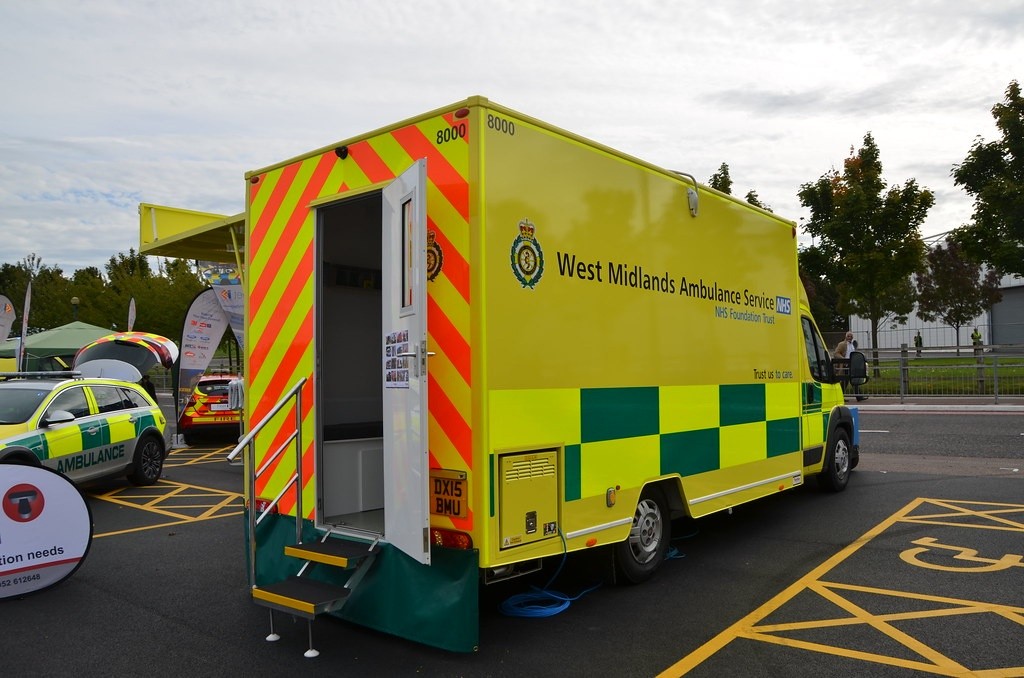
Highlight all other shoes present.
[857,397,868,401]
[844,399,849,402]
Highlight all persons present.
[914,332,923,357]
[970,328,983,358]
[142,374,158,405]
[834,331,868,403]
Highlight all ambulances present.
[181,375,243,443]
[0,371,174,486]
[137,95,870,660]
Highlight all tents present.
[0,320,124,379]
